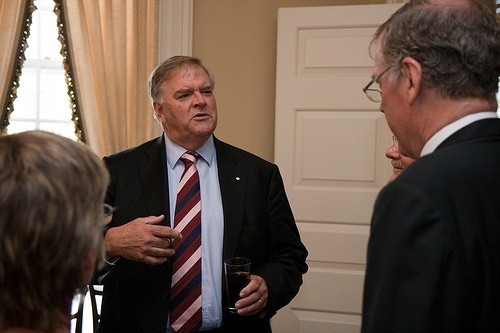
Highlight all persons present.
[385,133,417,176]
[96,56,308,333]
[0,129,114,333]
[361,0,500,333]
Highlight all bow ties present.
[165,152,203,333]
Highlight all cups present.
[224,257,251,314]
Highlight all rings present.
[166,237,173,248]
[258,299,263,305]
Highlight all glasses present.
[103,203,117,226]
[363,62,396,102]
